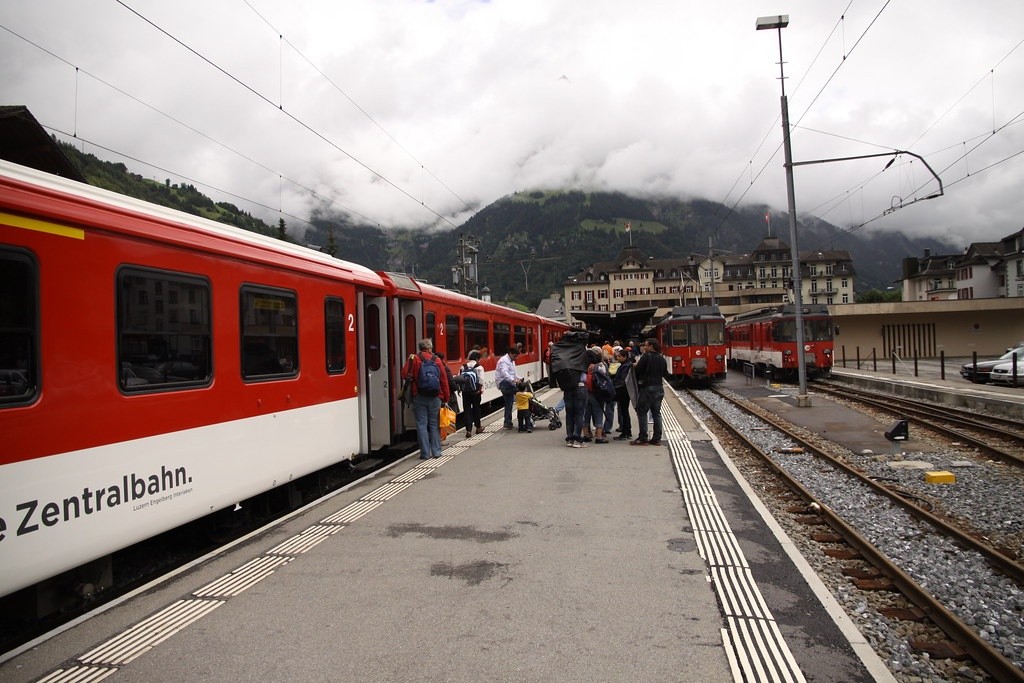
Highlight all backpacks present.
[460,363,483,397]
[417,354,441,398]
[592,364,616,403]
[543,347,551,363]
[554,365,581,391]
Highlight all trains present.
[0,158,579,618]
[642,303,728,384]
[724,302,841,382]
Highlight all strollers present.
[513,378,562,431]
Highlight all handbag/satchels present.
[398,354,414,405]
[439,402,457,433]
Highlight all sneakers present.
[567,440,573,446]
[573,440,591,448]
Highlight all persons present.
[515,382,533,433]
[458,351,485,438]
[551,327,593,449]
[400,338,450,460]
[467,340,531,359]
[613,349,635,441]
[590,339,641,365]
[635,342,657,425]
[494,347,522,430]
[434,351,456,448]
[580,346,611,444]
[544,341,554,384]
[629,339,668,447]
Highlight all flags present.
[765,212,769,222]
[625,223,631,232]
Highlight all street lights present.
[753,15,813,409]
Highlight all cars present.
[989,355,1024,385]
[959,343,1024,384]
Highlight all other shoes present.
[503,426,515,430]
[595,438,609,443]
[518,427,527,433]
[604,431,611,434]
[526,428,532,433]
[440,441,449,446]
[566,432,593,442]
[595,431,607,437]
[630,438,649,447]
[645,437,661,446]
[476,427,485,434]
[616,427,623,432]
[466,430,472,438]
[613,434,632,440]
